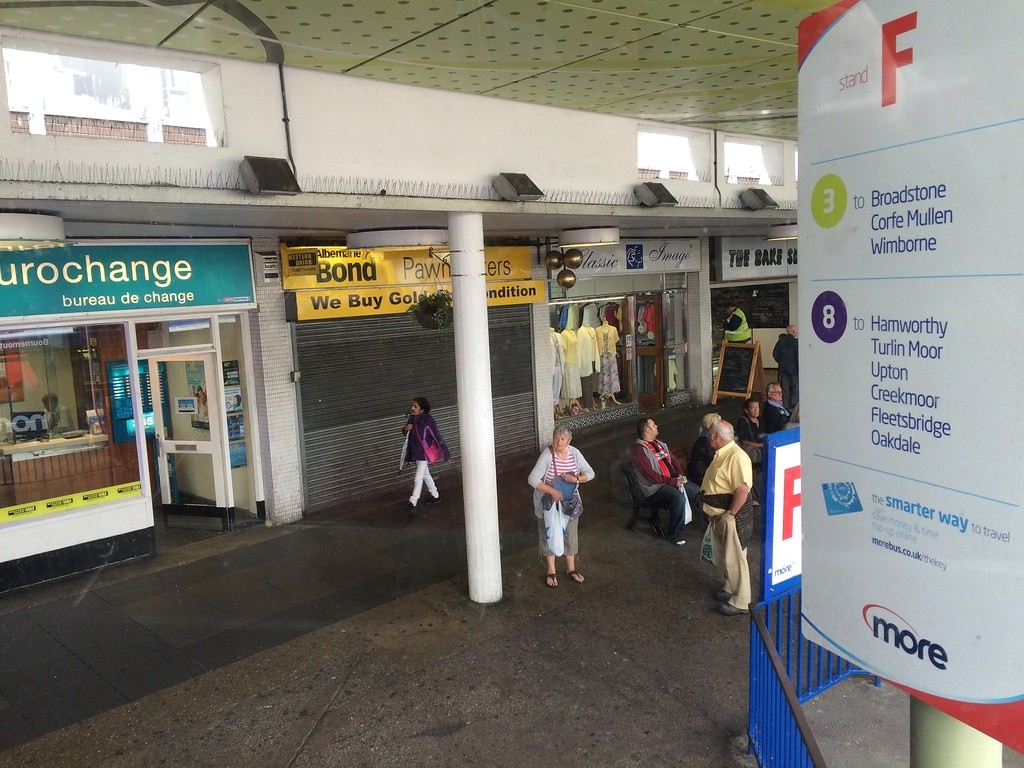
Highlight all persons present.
[551,320,622,418]
[632,417,703,546]
[190,385,209,423]
[691,383,799,506]
[718,301,753,345]
[528,424,595,588]
[695,419,756,615]
[772,325,798,412]
[401,398,452,512]
[227,395,241,410]
[41,393,71,434]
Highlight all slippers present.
[546,573,559,588]
[564,570,585,584]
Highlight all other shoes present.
[406,501,419,515]
[423,496,442,506]
[669,533,687,547]
[713,590,732,601]
[718,603,750,615]
[692,519,709,530]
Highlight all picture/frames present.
[176,398,198,414]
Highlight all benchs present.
[623,440,762,539]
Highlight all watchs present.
[576,477,580,484]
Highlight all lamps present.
[491,171,547,201]
[240,154,302,199]
[767,223,798,242]
[739,188,781,211]
[634,182,680,209]
[557,227,622,248]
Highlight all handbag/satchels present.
[679,485,692,525]
[542,501,570,557]
[699,522,715,566]
[541,471,584,521]
[424,441,442,465]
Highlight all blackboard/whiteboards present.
[713,340,765,397]
[710,282,790,329]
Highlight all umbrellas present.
[398,413,414,475]
